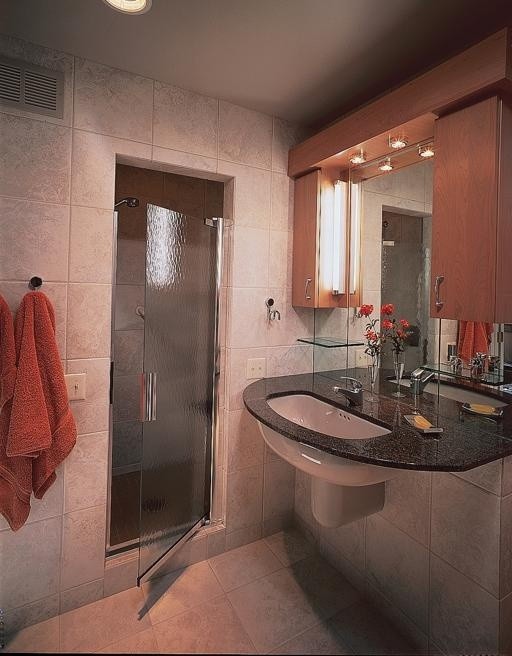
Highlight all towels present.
[0,293,78,532]
[454,320,494,364]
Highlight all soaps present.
[470,403,492,414]
[414,415,431,428]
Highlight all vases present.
[391,351,408,399]
[365,350,382,398]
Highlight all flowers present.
[356,305,393,389]
[381,305,410,390]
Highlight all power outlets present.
[248,358,266,379]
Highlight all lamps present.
[348,152,366,166]
[419,145,435,159]
[377,161,394,173]
[333,178,343,295]
[387,136,408,150]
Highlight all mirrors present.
[314,135,512,401]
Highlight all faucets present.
[333,376,364,406]
[409,361,435,395]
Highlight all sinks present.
[385,366,512,410]
[265,389,394,441]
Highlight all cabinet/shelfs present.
[293,170,348,307]
[427,95,512,323]
